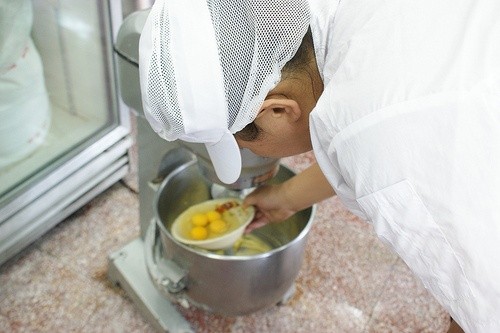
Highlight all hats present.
[138,0,311,185]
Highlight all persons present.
[137,0,496,332]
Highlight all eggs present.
[177,205,241,242]
